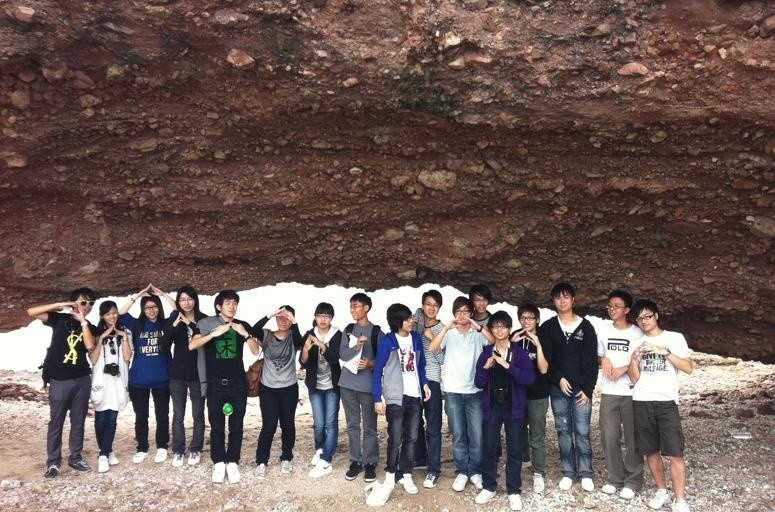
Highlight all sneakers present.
[522,461,532,468]
[559,477,573,490]
[132,451,149,464]
[211,462,226,483]
[647,489,671,509]
[452,473,468,491]
[280,460,293,477]
[671,498,690,512]
[43,464,59,480]
[413,460,427,470]
[619,486,636,499]
[311,448,323,465]
[386,471,396,489]
[226,462,241,484]
[254,463,266,481]
[109,452,120,466]
[154,448,168,463]
[345,463,362,481]
[309,460,332,478]
[364,463,377,482]
[423,473,440,488]
[98,456,109,473]
[507,494,523,510]
[398,473,419,495]
[172,452,184,468]
[187,451,201,466]
[68,459,91,473]
[601,484,617,494]
[470,474,483,489]
[532,472,545,494]
[581,478,594,492]
[475,489,497,504]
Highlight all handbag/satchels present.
[244,358,264,397]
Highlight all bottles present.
[223,403,233,416]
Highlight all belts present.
[211,378,244,385]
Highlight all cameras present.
[104,363,120,375]
[492,385,509,403]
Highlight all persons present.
[300,282,692,512]
[27,285,259,484]
[252,305,304,477]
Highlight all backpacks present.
[42,347,50,385]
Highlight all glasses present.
[349,305,364,310]
[74,300,95,306]
[520,317,536,321]
[455,310,470,314]
[178,298,194,303]
[405,315,412,322]
[143,305,159,311]
[489,325,506,329]
[635,313,654,322]
[424,303,439,308]
[606,304,627,310]
[109,341,116,355]
[315,315,330,318]
